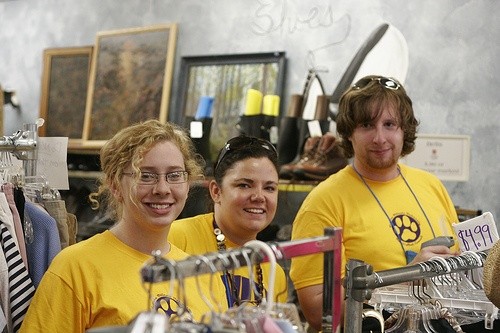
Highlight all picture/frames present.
[38,24,177,148]
[176,51,287,171]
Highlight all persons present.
[168,135,289,325]
[15,120,229,333]
[289,75,500,333]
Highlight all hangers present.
[0,135,62,201]
[353,248,499,333]
[88,240,295,333]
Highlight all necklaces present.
[213,221,263,306]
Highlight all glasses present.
[122,170,189,185]
[356,76,400,91]
[214,138,278,170]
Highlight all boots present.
[287,133,350,180]
[277,136,322,180]
